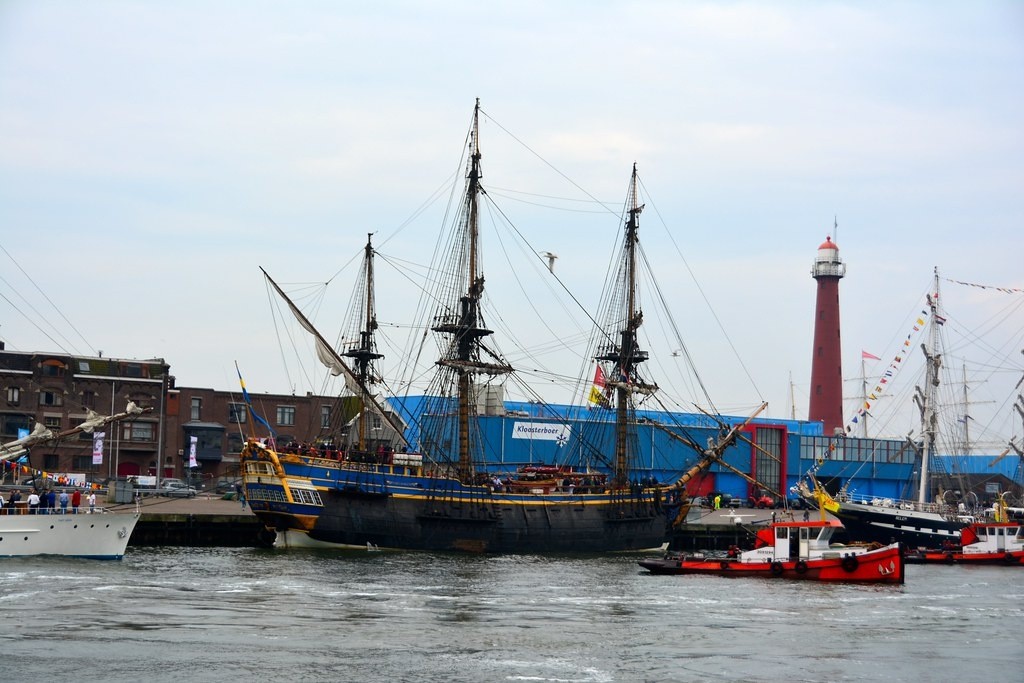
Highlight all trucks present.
[36,472,87,486]
[128,473,163,489]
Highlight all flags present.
[594,364,614,398]
[590,387,611,410]
[0,457,106,489]
[790,278,1023,492]
[620,363,631,384]
[92,431,105,464]
[189,436,198,468]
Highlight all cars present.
[23,475,42,485]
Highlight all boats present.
[636,521,907,585]
[852,521,1024,568]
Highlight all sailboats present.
[235,98,770,552]
[0,363,155,562]
[789,264,1024,547]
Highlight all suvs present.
[160,477,197,498]
[216,480,232,494]
[101,476,127,488]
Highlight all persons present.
[267,435,669,494]
[0,486,97,514]
[803,508,810,522]
[729,507,736,522]
[714,494,720,510]
[241,491,248,511]
[726,544,740,559]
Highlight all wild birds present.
[538,250,557,274]
[669,349,682,357]
